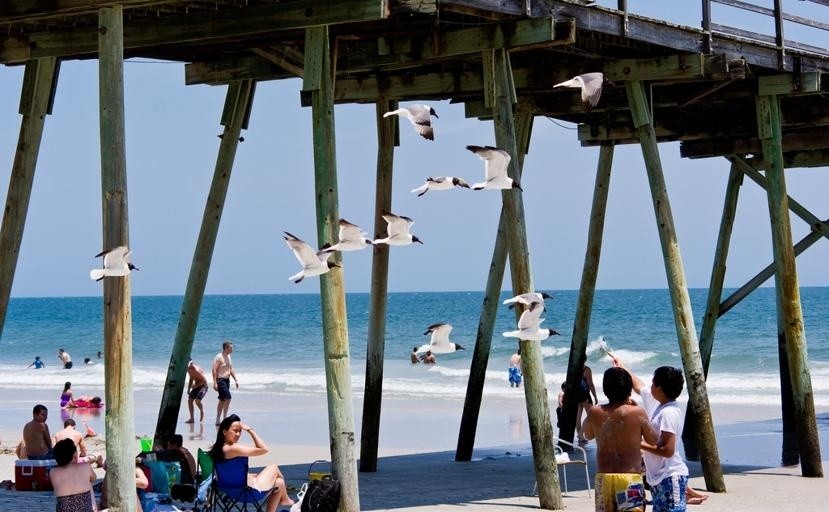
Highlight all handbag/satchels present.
[301,475,340,512]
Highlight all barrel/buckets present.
[141,439,151,452]
[308,460,332,481]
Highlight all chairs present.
[210,458,280,512]
[532,435,593,501]
[195,448,241,511]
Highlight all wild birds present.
[553,71,616,107]
[502,306,561,342]
[413,324,466,361]
[322,218,377,252]
[89,245,140,282]
[465,145,523,192]
[373,209,423,246]
[503,292,553,313]
[280,230,342,286]
[410,176,470,197]
[383,104,439,141]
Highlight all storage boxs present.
[14,460,56,492]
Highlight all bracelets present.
[246,427,252,434]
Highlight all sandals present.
[156,497,171,504]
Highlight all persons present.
[507,349,523,387]
[557,348,690,511]
[211,340,239,426]
[22,348,196,511]
[186,359,208,424]
[413,348,435,364]
[206,414,295,512]
